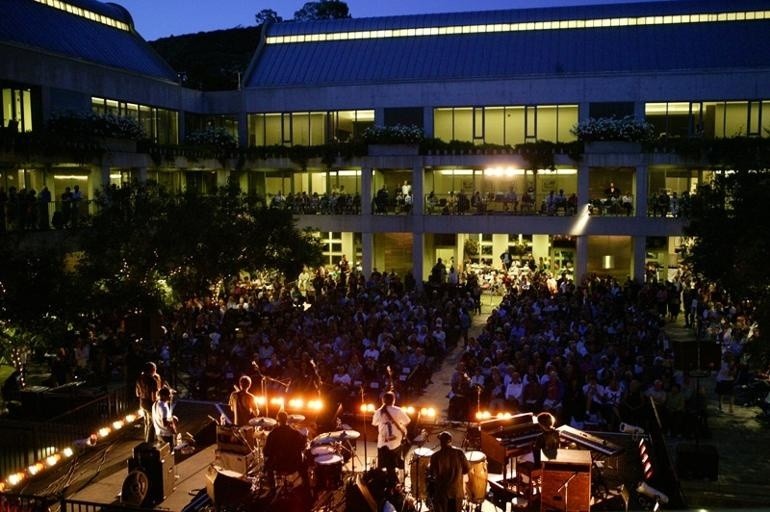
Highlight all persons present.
[537,413,561,460]
[371,392,411,470]
[52,255,770,431]
[61,185,82,210]
[136,362,161,443]
[275,184,361,216]
[7,186,51,229]
[590,182,634,217]
[263,413,309,495]
[376,181,412,215]
[648,189,690,218]
[150,386,177,453]
[428,185,578,216]
[427,432,469,512]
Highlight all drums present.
[410,448,433,500]
[465,451,487,504]
[315,455,342,489]
[309,445,333,486]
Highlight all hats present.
[708,301,723,307]
[437,431,453,443]
[284,283,471,331]
[482,334,663,371]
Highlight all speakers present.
[127,452,175,502]
[672,339,722,371]
[540,449,592,512]
[205,464,252,503]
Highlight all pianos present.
[479,412,546,482]
[556,426,623,456]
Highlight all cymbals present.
[288,414,305,423]
[249,417,277,426]
[338,430,359,438]
[316,433,338,443]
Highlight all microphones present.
[387,365,392,375]
[252,360,259,368]
[310,359,316,367]
[463,373,472,383]
